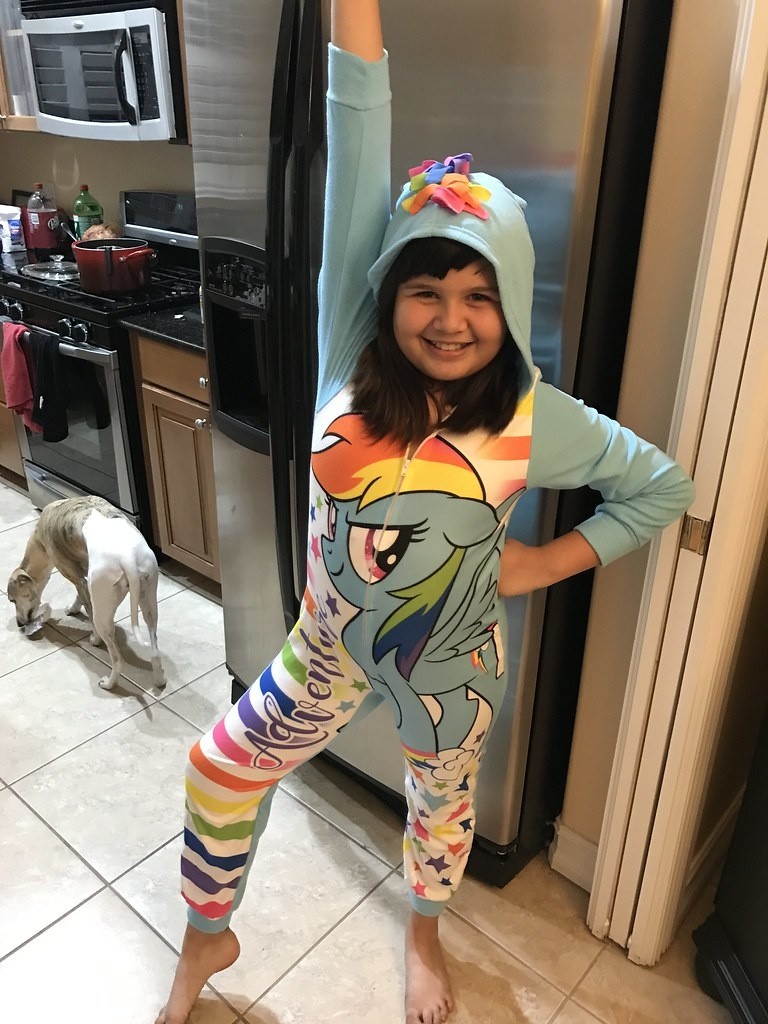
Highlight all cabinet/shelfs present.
[1,325,24,479]
[138,333,222,586]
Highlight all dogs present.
[7,495,168,690]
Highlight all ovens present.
[1,295,154,555]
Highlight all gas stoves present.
[0,253,200,327]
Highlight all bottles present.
[26,183,64,264]
[73,184,103,242]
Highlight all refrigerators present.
[181,0,672,894]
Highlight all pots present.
[71,236,155,293]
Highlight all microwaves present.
[19,6,186,141]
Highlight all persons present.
[155,0,698,1024]
